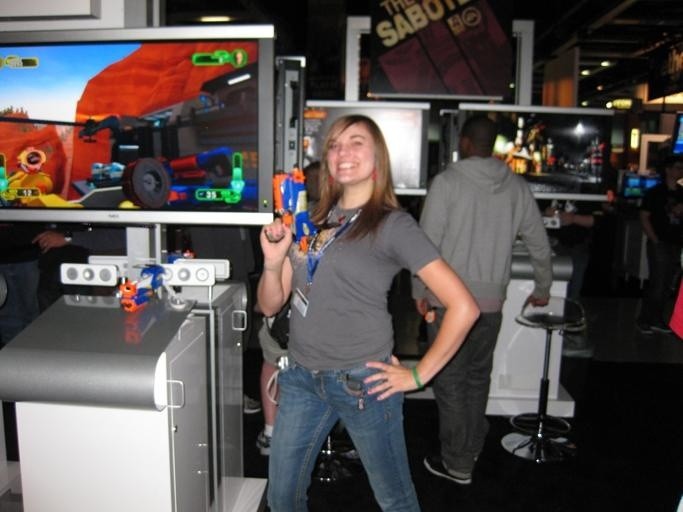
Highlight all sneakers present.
[256,432,272,456]
[636,319,651,334]
[652,320,672,333]
[424,455,472,484]
[243,394,261,413]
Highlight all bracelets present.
[411,364,423,391]
[63,231,72,245]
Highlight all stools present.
[499,293,589,465]
[267,293,362,484]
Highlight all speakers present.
[173,258,230,281]
[540,216,560,227]
[87,255,129,277]
[159,265,215,287]
[59,263,119,287]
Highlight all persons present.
[636,153,683,335]
[0,222,41,330]
[257,161,320,456]
[190,226,260,415]
[31,223,126,256]
[411,112,552,481]
[544,201,609,295]
[258,114,481,512]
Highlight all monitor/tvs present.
[671,112,683,158]
[458,103,615,202]
[0,25,275,224]
[301,100,432,195]
[273,57,306,214]
